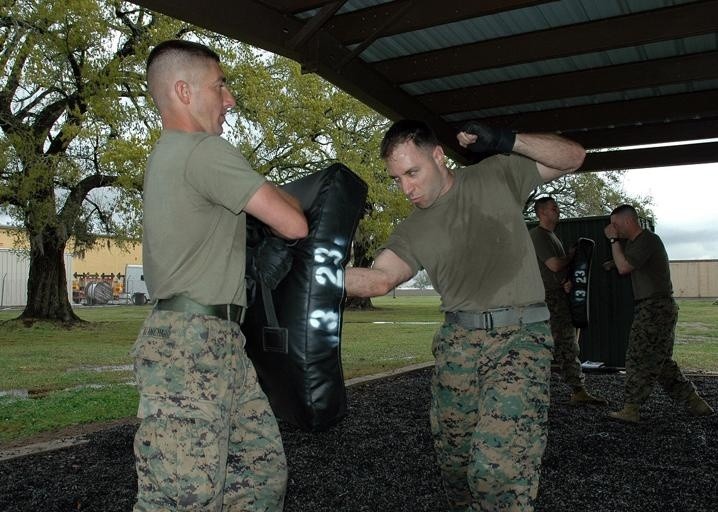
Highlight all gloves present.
[252,237,294,291]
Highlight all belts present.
[157,297,243,324]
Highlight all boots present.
[686,390,714,416]
[609,404,642,422]
[571,384,608,405]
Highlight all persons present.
[131,40,309,512]
[343,120,585,512]
[527,197,610,408]
[604,205,713,425]
